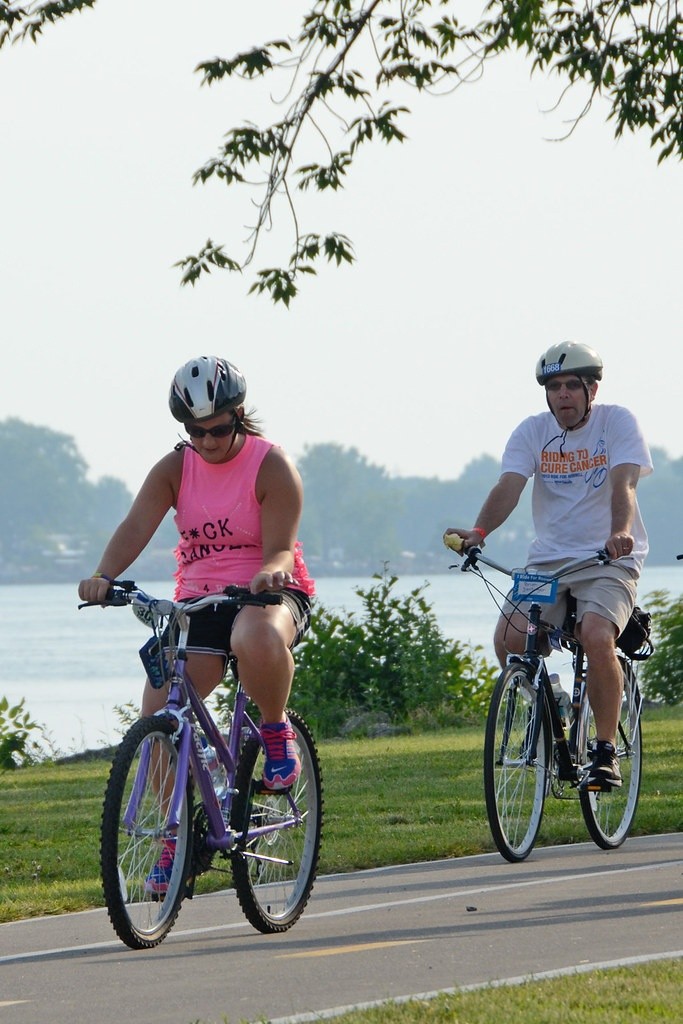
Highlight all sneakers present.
[143,832,178,895]
[518,705,537,765]
[587,737,622,787]
[259,710,302,790]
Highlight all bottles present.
[548,673,575,730]
[200,737,228,797]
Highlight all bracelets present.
[91,573,113,582]
[471,528,486,541]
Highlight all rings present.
[623,547,629,550]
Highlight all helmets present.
[169,356,247,424]
[536,345,603,386]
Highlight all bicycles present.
[77,580,326,951]
[447,538,647,864]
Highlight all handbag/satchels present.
[616,605,655,661]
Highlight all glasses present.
[184,413,236,439]
[545,379,590,392]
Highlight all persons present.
[79,357,318,895]
[442,340,654,789]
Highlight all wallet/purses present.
[139,636,172,690]
